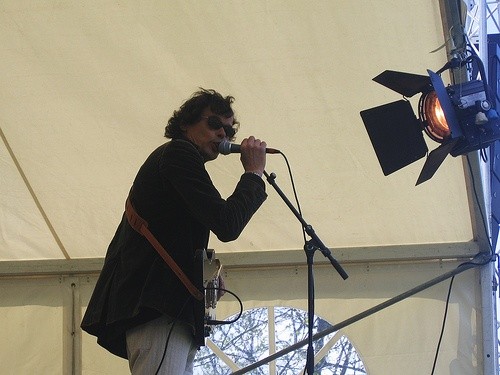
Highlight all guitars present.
[192,247,224,346]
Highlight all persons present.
[79,87,268,375]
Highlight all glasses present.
[202,115,235,138]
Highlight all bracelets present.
[244,170,260,178]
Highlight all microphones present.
[218,141,280,155]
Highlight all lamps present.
[359,55,500,186]
